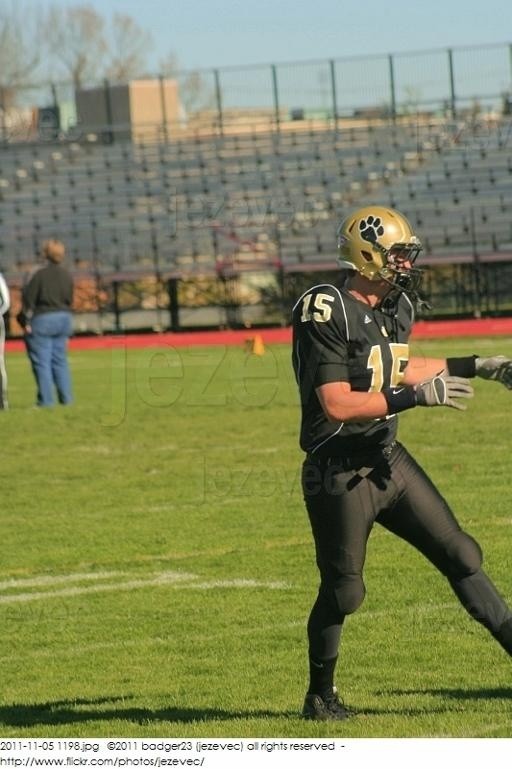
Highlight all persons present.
[0,272,12,409]
[292,205,511,723]
[13,239,76,407]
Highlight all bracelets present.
[379,384,417,415]
[446,354,480,379]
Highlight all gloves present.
[414,367,474,411]
[474,355,512,389]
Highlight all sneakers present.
[301,687,357,721]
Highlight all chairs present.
[0,115,510,274]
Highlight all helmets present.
[336,206,425,293]
